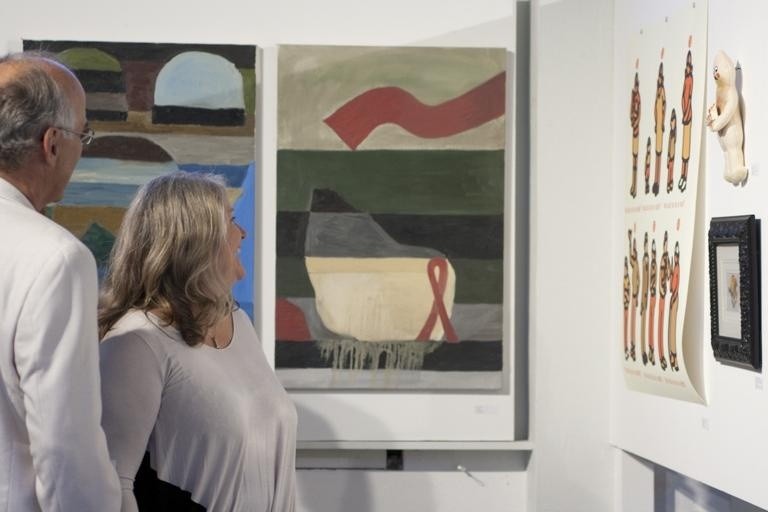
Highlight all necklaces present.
[204,325,220,350]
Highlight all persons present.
[97,174,300,512]
[1,49,122,512]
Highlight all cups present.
[54,126,95,147]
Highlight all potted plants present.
[708,213,762,371]
